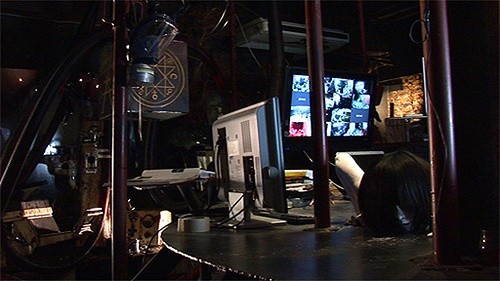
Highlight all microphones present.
[424,7,430,33]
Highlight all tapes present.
[177,216,210,232]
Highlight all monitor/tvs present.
[212,97,288,215]
[281,64,379,150]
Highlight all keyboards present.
[267,211,315,225]
